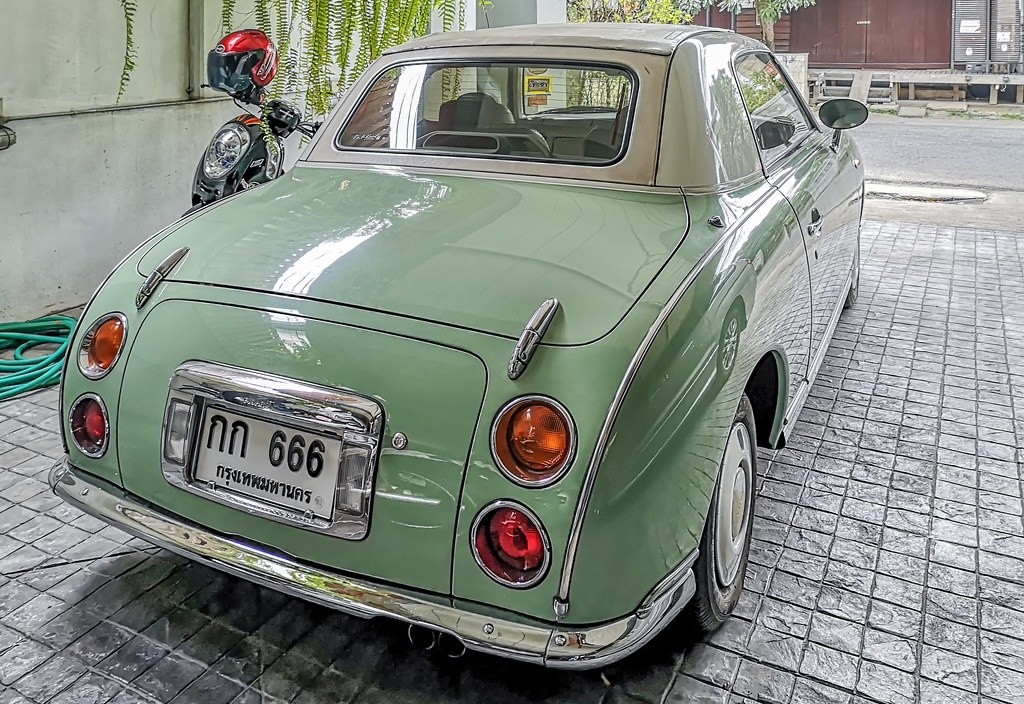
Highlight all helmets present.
[215,29,279,87]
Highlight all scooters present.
[183,75,329,218]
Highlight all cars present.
[45,22,870,672]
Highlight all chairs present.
[585,106,629,159]
[440,92,527,151]
